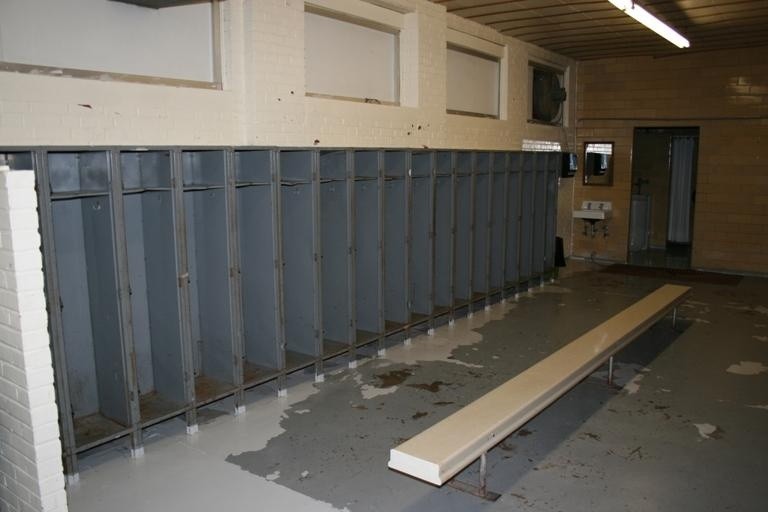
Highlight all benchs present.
[388,283,692,502]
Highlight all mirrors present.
[583,141,614,186]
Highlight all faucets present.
[586,203,592,209]
[598,203,604,209]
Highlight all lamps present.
[610,0,691,49]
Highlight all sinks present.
[572,208,612,219]
